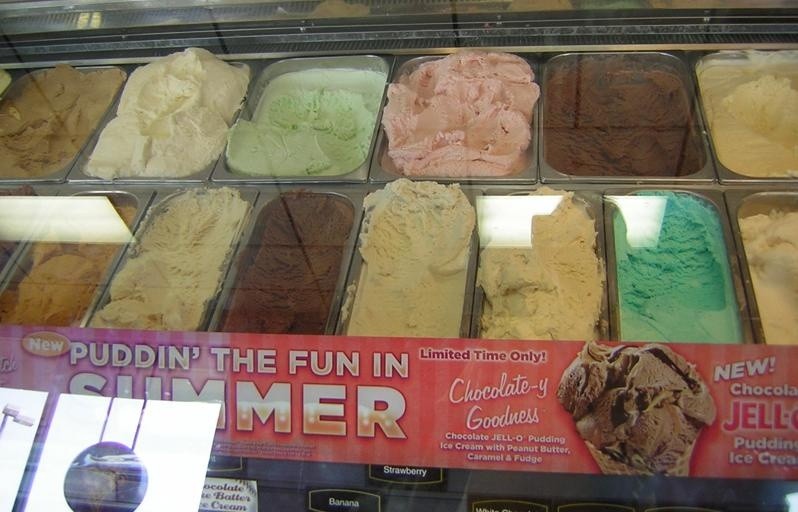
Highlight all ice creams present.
[555,339,716,477]
